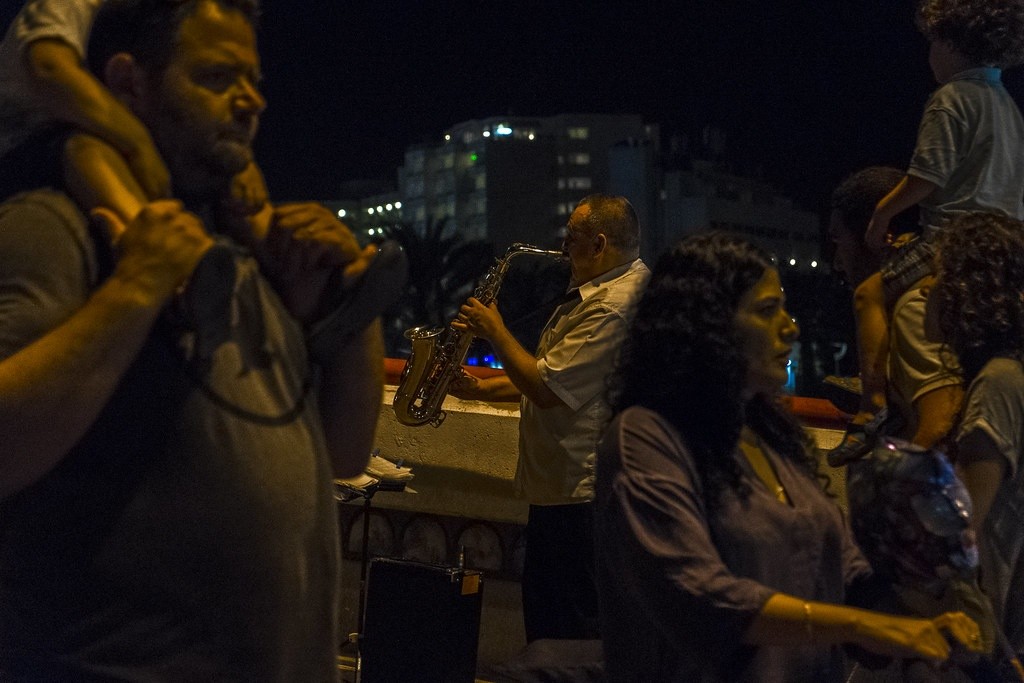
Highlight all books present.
[330,453,418,498]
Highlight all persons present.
[446,0,1024,683]
[0,0,382,683]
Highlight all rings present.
[968,634,979,644]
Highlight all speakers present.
[357,557,484,683]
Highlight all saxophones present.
[390,242,572,428]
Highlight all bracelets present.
[804,599,814,648]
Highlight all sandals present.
[821,375,869,415]
[827,407,890,468]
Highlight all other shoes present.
[301,241,407,357]
[166,244,238,371]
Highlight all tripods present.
[331,481,407,683]
